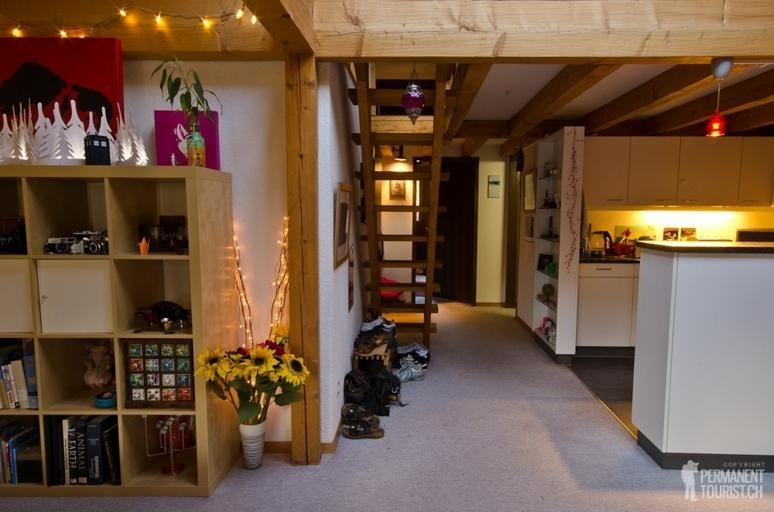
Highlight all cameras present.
[72,231,102,252]
[47,238,76,254]
[84,239,108,255]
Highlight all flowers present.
[194,323,310,424]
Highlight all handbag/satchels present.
[344,367,400,409]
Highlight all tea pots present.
[588,230,613,257]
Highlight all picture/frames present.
[390,180,405,200]
[333,182,354,271]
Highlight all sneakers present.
[392,342,430,383]
[354,316,397,354]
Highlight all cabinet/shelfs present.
[577,264,639,347]
[584,137,774,206]
[0,165,241,496]
[535,171,560,314]
[516,240,535,330]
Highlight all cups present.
[164,321,175,333]
[176,316,191,330]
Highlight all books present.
[0,338,39,410]
[0,415,121,488]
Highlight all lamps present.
[12,3,257,37]
[706,56,735,137]
[392,145,407,161]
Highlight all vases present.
[238,419,272,468]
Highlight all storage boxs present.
[0,37,125,141]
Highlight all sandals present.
[340,403,384,439]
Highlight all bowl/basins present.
[93,393,116,408]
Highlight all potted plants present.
[151,55,223,167]
